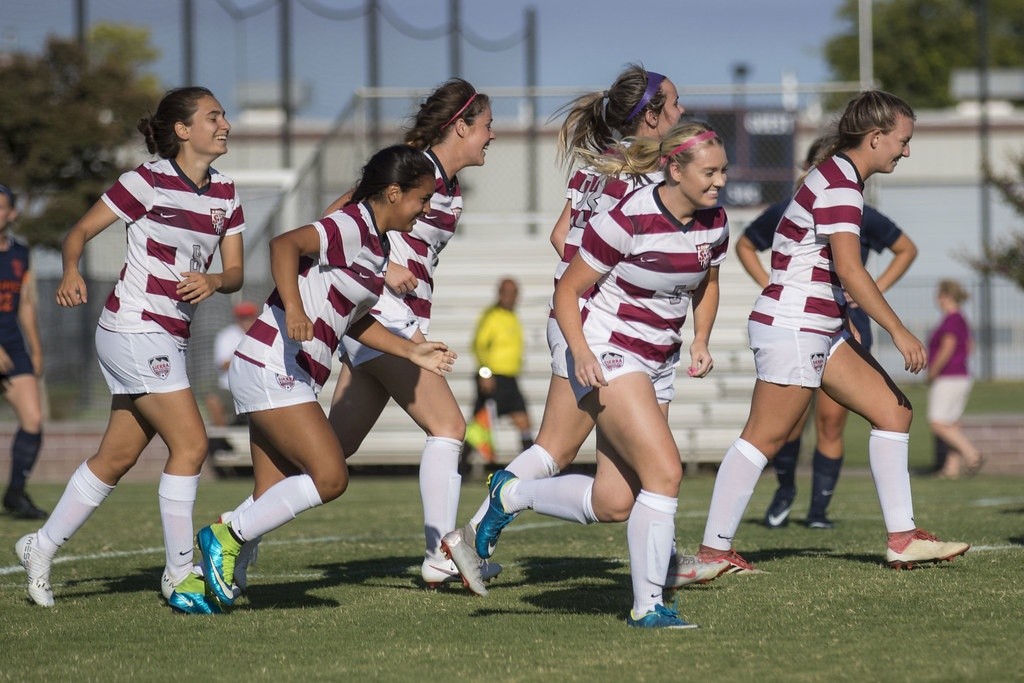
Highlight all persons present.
[477,118,729,633]
[698,90,969,570]
[738,137,916,529]
[923,281,983,480]
[197,145,457,609]
[439,65,731,596]
[205,303,261,426]
[323,77,501,589]
[15,86,246,609]
[0,184,48,519]
[455,278,535,473]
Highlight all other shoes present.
[764,485,796,529]
[13,533,55,609]
[3,491,47,519]
[160,565,206,600]
[806,508,834,531]
[927,469,960,483]
[965,453,985,478]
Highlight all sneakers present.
[169,573,253,614]
[662,539,731,591]
[422,556,502,589]
[699,549,772,576]
[887,528,971,569]
[473,469,518,559]
[626,595,698,630]
[439,526,489,597]
[195,523,242,606]
[218,511,259,592]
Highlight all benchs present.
[198,207,771,467]
